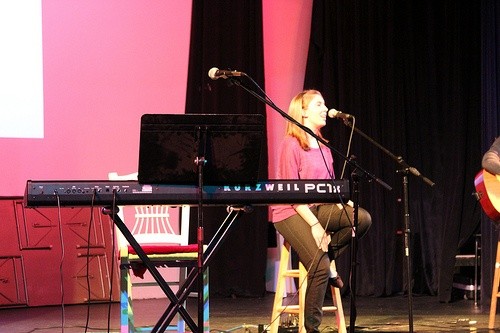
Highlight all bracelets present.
[310,221,320,227]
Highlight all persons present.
[269,90,372,333]
[481,136,500,175]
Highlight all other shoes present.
[329,274,343,287]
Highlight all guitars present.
[474,168,500,220]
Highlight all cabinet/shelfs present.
[453,240,481,310]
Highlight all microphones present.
[328,109,353,118]
[208,67,243,80]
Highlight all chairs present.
[109,171,210,333]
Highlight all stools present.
[270,232,347,333]
[488,241,500,329]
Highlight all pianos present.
[22,178,350,208]
